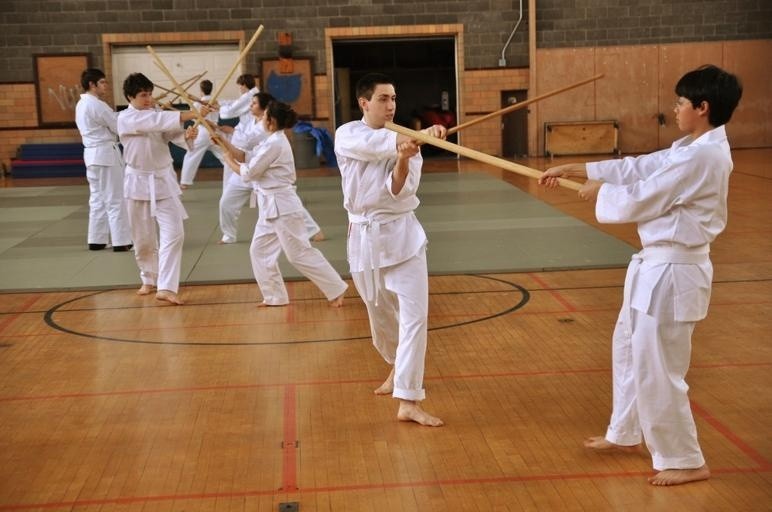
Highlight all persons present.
[209,98,350,309]
[216,73,260,194]
[218,91,327,243]
[333,67,449,428]
[537,64,745,487]
[178,79,231,189]
[74,67,134,252]
[501,97,526,160]
[115,72,200,306]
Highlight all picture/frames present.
[31,52,92,129]
[260,56,316,121]
